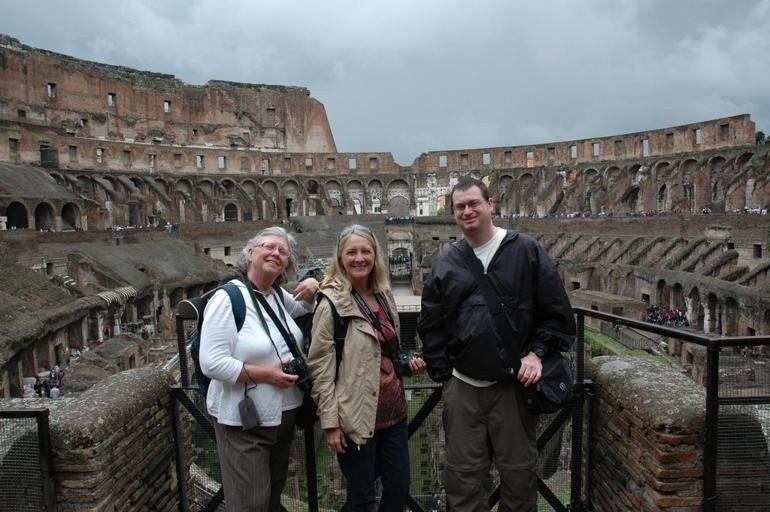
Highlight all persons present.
[643,301,690,328]
[307,223,430,512]
[33,304,165,398]
[414,177,578,510]
[197,226,321,510]
[106,220,181,241]
[491,205,770,221]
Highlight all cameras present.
[281,355,310,383]
[393,351,421,377]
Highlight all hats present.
[177,284,265,331]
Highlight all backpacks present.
[189,280,285,398]
[302,292,401,389]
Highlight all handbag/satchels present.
[515,351,572,415]
[294,395,320,430]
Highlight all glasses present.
[342,248,373,256]
[453,198,486,211]
[254,242,290,257]
